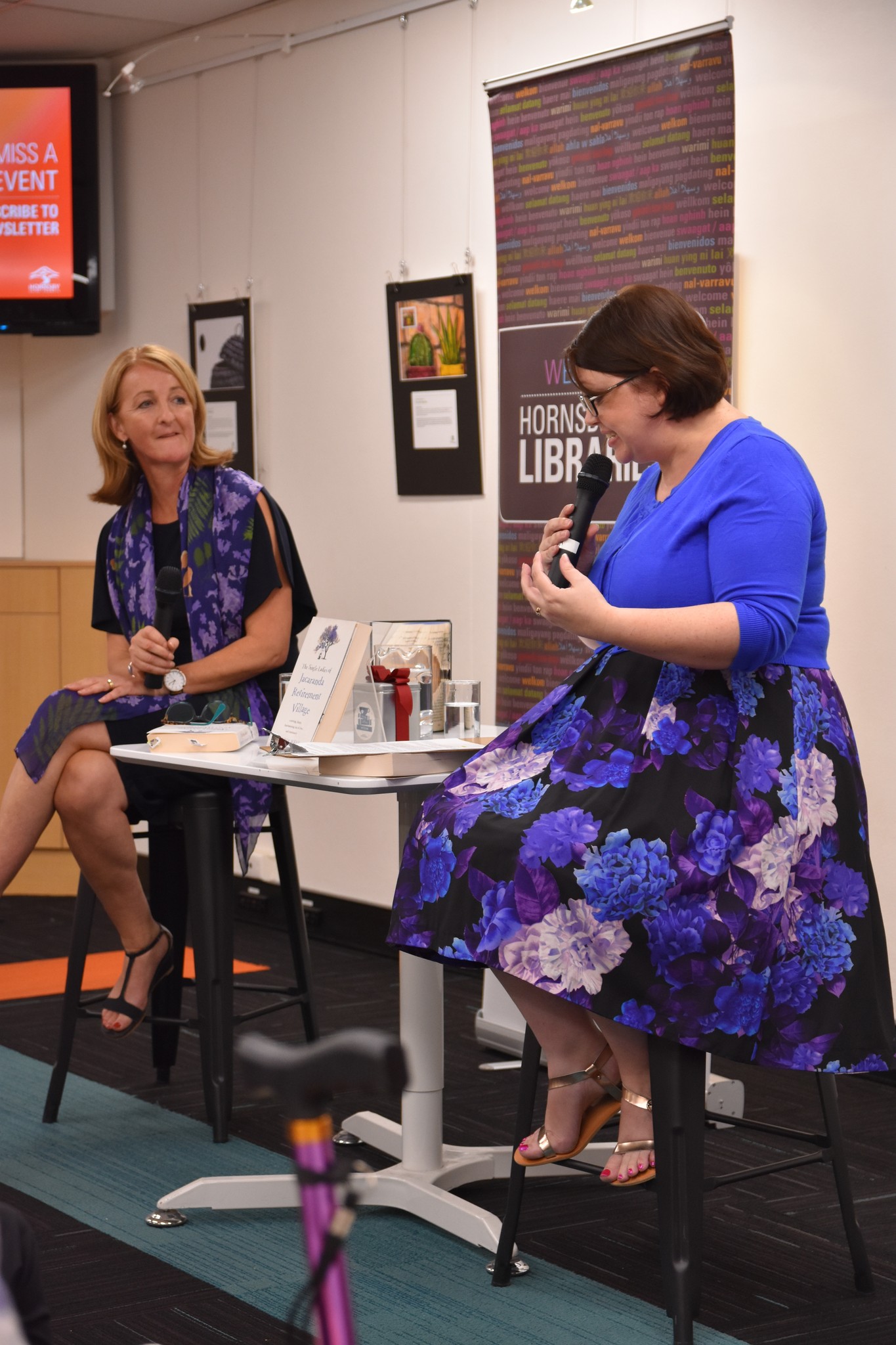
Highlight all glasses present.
[578,370,642,418]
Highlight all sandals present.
[514,1042,622,1166]
[609,1084,656,1186]
[101,921,174,1041]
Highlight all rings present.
[107,679,114,690]
[536,608,541,616]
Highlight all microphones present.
[546,453,613,589]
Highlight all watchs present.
[163,666,186,696]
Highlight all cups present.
[444,681,482,738]
[279,673,295,707]
[375,644,434,740]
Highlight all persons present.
[388,283,896,1186]
[1,345,319,1036]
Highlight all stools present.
[43,775,326,1146]
[492,1045,872,1344]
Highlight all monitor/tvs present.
[0,62,102,335]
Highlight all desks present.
[108,728,618,1277]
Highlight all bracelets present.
[127,661,135,677]
